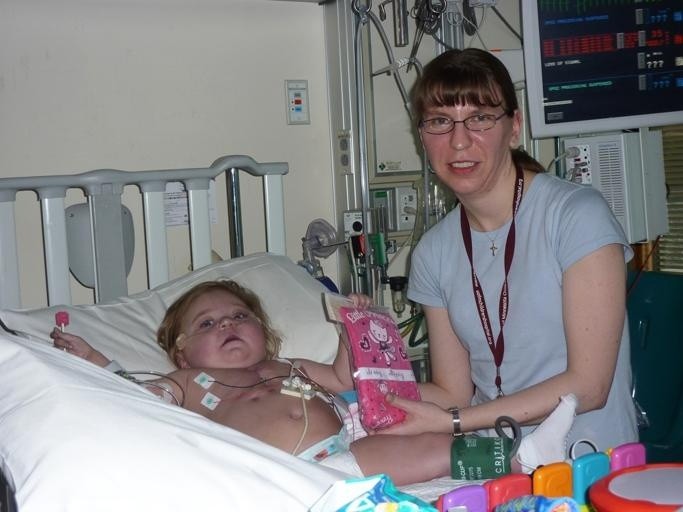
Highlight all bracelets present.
[446,405,463,439]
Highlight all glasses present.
[416,105,511,133]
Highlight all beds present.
[1,154,628,511]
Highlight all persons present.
[48,282,576,487]
[362,47,639,460]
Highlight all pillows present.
[1,254,341,382]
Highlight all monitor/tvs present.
[520,0,683,139]
